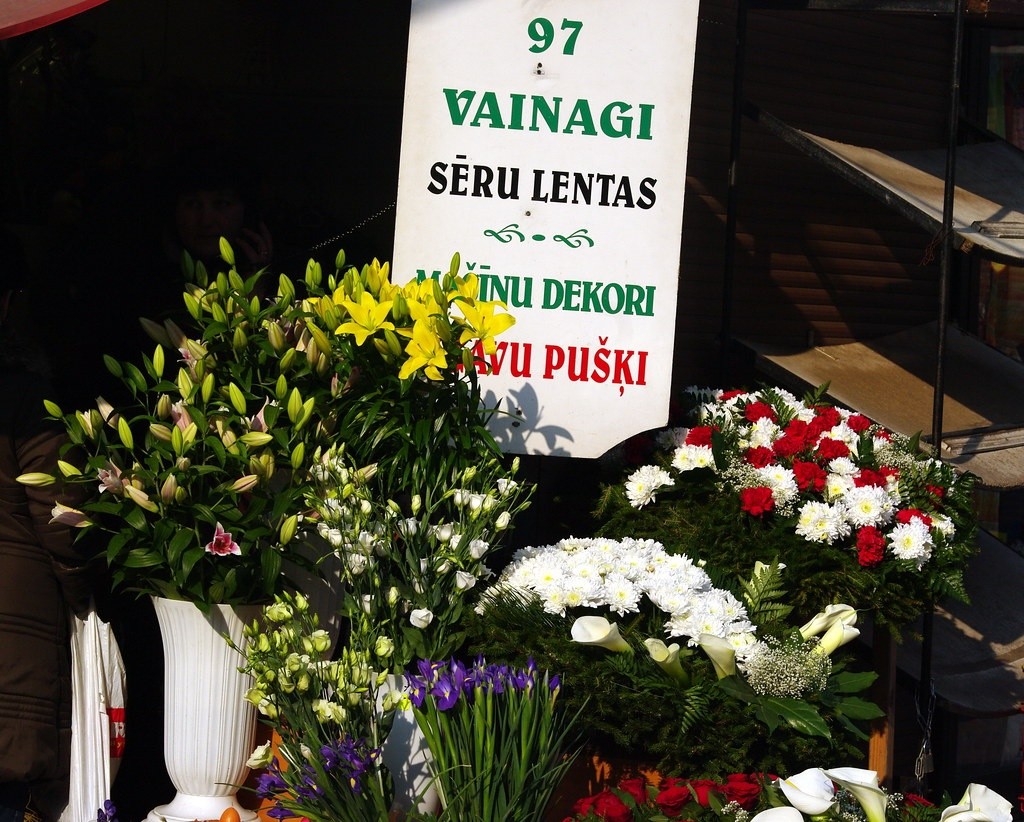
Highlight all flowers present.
[13,233,982,822]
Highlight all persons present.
[121,141,306,360]
[0,226,93,821]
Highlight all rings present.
[259,249,268,255]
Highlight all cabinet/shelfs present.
[710,0,1024,811]
